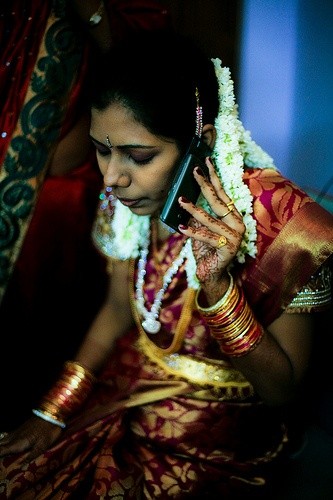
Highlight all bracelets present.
[193,272,265,358]
[32,360,100,429]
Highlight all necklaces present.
[123,240,193,358]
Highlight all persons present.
[0,23,333,500]
[75,1,110,52]
[15,108,104,314]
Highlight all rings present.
[222,199,236,218]
[216,235,227,250]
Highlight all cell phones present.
[160,135,214,233]
